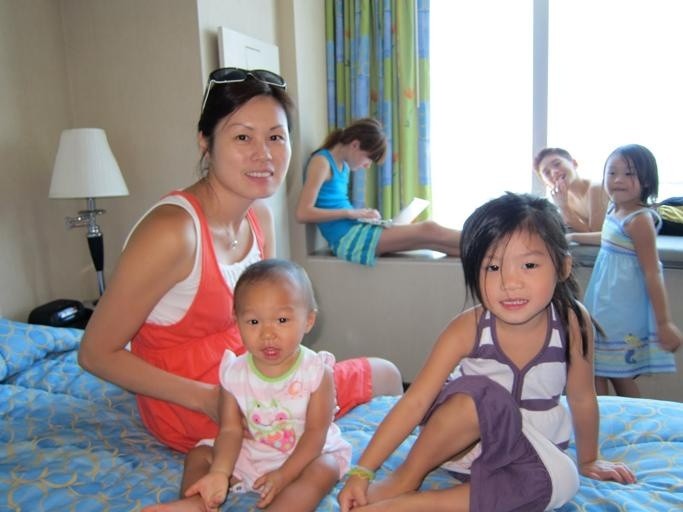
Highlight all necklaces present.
[225,220,242,251]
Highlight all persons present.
[77,67,405,453]
[337,192,636,512]
[533,147,682,236]
[140,257,352,512]
[564,143,682,396]
[295,117,462,266]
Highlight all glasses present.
[202,67,288,118]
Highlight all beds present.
[0,317,683,512]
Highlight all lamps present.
[49,127,131,296]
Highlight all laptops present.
[357,197,430,228]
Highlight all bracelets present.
[345,464,375,483]
